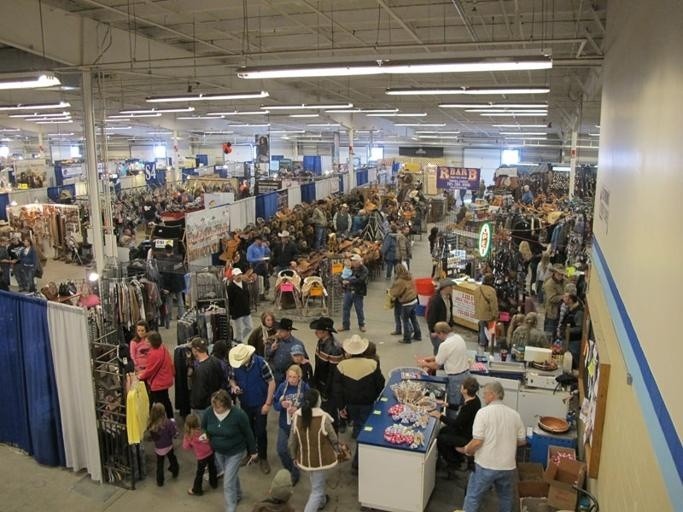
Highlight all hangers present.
[212,301,225,313]
[111,276,130,289]
[130,276,139,287]
[135,276,144,287]
[204,300,220,311]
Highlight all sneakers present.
[238,456,251,467]
[359,326,367,333]
[337,325,344,332]
[260,462,271,474]
[397,338,411,344]
[413,336,422,340]
[390,331,401,335]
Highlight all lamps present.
[324,97,401,113]
[145,78,270,104]
[438,95,549,108]
[259,78,355,110]
[385,74,550,95]
[0,93,71,110]
[0,0,61,88]
[236,0,553,80]
[119,77,194,114]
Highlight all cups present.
[500,349,508,361]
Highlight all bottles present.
[511,343,516,358]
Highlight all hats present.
[186,336,208,349]
[268,469,294,503]
[254,233,266,240]
[290,344,306,357]
[228,344,256,370]
[434,321,452,333]
[438,278,457,288]
[278,231,291,238]
[340,203,349,208]
[231,268,243,276]
[342,333,370,355]
[548,263,568,275]
[309,316,337,334]
[271,318,298,331]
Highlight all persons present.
[0,180,589,511]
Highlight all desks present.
[355,375,449,512]
[472,370,573,432]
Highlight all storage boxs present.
[520,497,556,512]
[547,445,576,465]
[516,463,548,496]
[544,458,586,511]
[526,426,578,471]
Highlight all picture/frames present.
[577,298,611,480]
